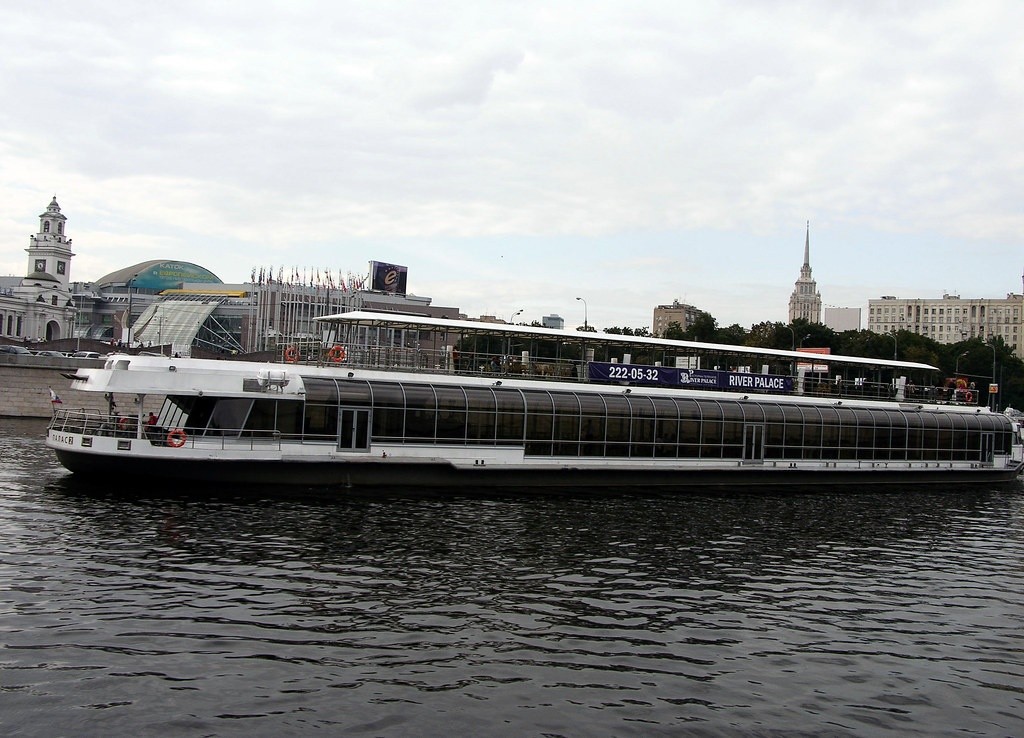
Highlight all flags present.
[49,388,61,404]
[251,264,364,294]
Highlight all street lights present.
[885,330,897,396]
[576,297,588,332]
[985,342,996,413]
[125,272,139,351]
[783,325,794,376]
[799,333,810,351]
[956,350,970,385]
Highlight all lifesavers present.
[330,346,345,362]
[167,429,187,447]
[965,392,972,402]
[285,347,299,361]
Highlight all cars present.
[0,344,167,360]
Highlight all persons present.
[146,411,157,431]
[905,379,976,403]
[22,336,48,349]
[1004,403,1014,416]
[110,338,153,347]
[452,344,578,379]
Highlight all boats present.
[39,307,1024,493]
